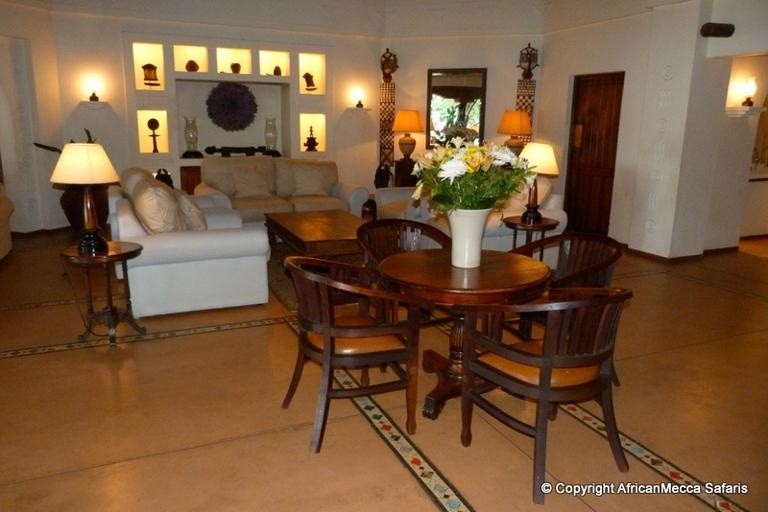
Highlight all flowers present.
[411,136,536,219]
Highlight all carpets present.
[267,243,364,312]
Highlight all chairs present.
[461,287,634,504]
[280,254,420,454]
[506,232,624,386]
[106,184,232,279]
[358,219,452,373]
[115,199,269,320]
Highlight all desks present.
[263,209,369,257]
[378,248,550,420]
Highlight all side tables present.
[61,241,146,343]
[504,216,559,262]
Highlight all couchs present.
[369,187,568,271]
[194,156,369,242]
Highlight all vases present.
[446,207,492,269]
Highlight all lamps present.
[392,110,425,161]
[379,48,399,168]
[742,76,758,106]
[496,110,533,156]
[51,143,121,254]
[515,43,539,146]
[515,142,561,222]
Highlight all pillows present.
[230,164,271,198]
[120,168,154,199]
[134,178,207,235]
[275,161,335,196]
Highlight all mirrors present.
[426,68,487,150]
